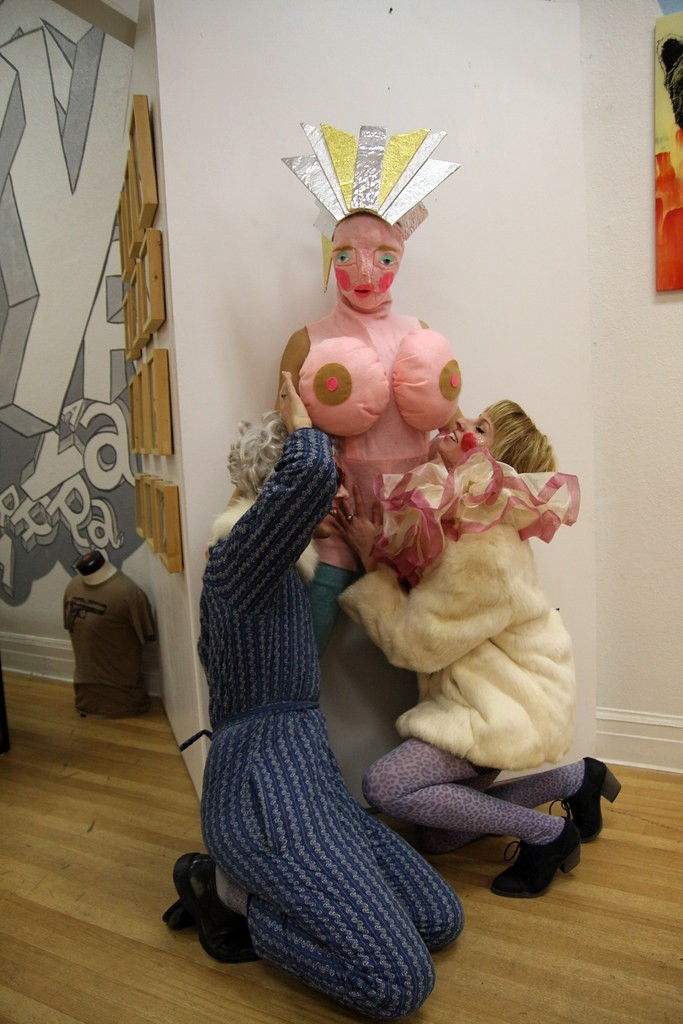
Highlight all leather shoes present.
[173,852,259,962]
[162,896,192,930]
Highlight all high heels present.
[548,757,621,842]
[491,816,581,898]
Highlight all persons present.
[57,551,158,720]
[332,398,621,896]
[162,367,465,1022]
[205,214,463,664]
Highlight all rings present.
[281,394,286,398]
[346,513,356,521]
